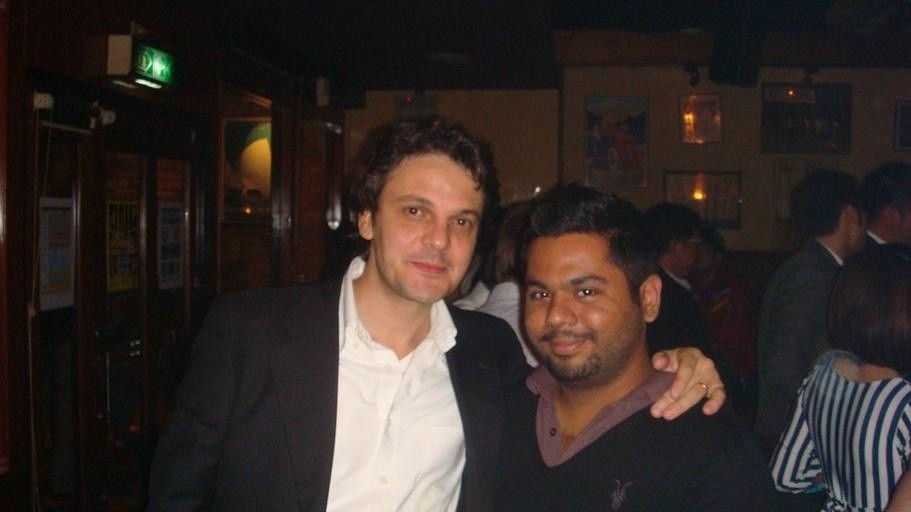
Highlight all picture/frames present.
[581,95,649,191]
[217,116,272,226]
[678,93,724,144]
[894,100,911,152]
[663,170,741,229]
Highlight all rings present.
[696,380,710,396]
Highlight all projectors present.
[391,94,439,117]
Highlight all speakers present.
[708,19,763,89]
[327,77,367,111]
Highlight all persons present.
[453,197,541,373]
[621,198,715,373]
[768,241,910,512]
[748,163,870,512]
[145,111,729,512]
[489,181,770,511]
[856,159,911,250]
[688,227,763,430]
[447,207,506,312]
